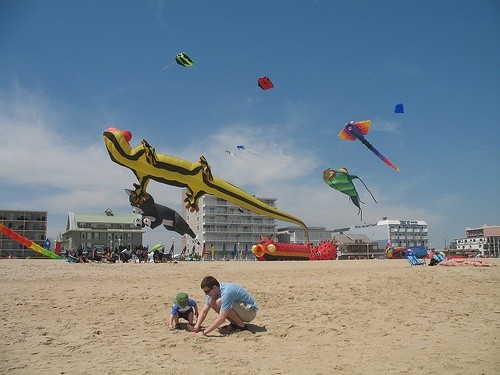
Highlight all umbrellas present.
[149,243,165,253]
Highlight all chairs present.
[407,256,426,266]
[433,254,449,265]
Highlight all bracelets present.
[202,330,206,335]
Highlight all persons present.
[169,291,198,331]
[193,276,259,338]
[69,243,148,264]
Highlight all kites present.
[395,104,403,114]
[322,166,377,221]
[256,76,274,92]
[176,52,194,68]
[124,184,197,238]
[336,120,400,172]
[102,127,308,240]
[224,150,239,157]
[237,145,248,153]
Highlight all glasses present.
[203,286,214,294]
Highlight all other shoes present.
[230,324,247,331]
[229,322,236,329]
[186,323,193,331]
[175,324,181,330]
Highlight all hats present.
[173,291,188,308]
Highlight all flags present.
[56,243,60,254]
[43,239,51,249]
[169,244,249,261]
